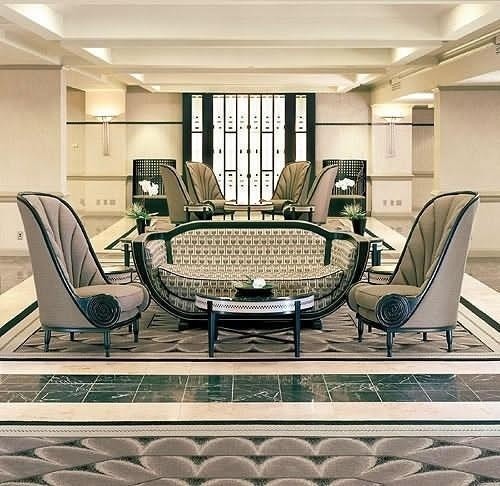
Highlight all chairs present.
[158,161,339,227]
[347,191,480,357]
[18,192,150,357]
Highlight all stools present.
[368,264,398,336]
[102,266,136,333]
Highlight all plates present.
[234,284,274,294]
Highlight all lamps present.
[383,116,404,124]
[96,115,114,122]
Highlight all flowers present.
[335,178,365,217]
[125,180,159,219]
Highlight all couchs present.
[131,220,370,330]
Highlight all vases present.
[136,219,146,236]
[353,217,365,237]
